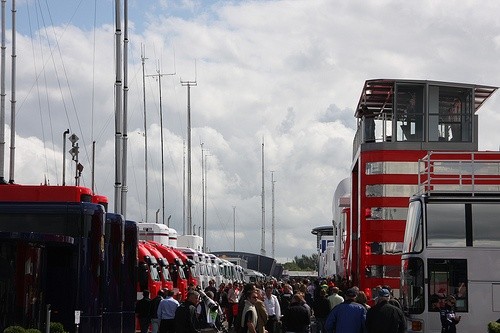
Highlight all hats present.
[346,289,356,297]
[378,289,389,298]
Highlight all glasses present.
[449,300,455,304]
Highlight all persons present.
[188,283,194,291]
[136,290,151,333]
[195,280,253,333]
[150,288,180,333]
[439,295,461,333]
[174,291,200,333]
[365,286,407,333]
[241,291,258,333]
[318,276,371,333]
[256,279,332,333]
